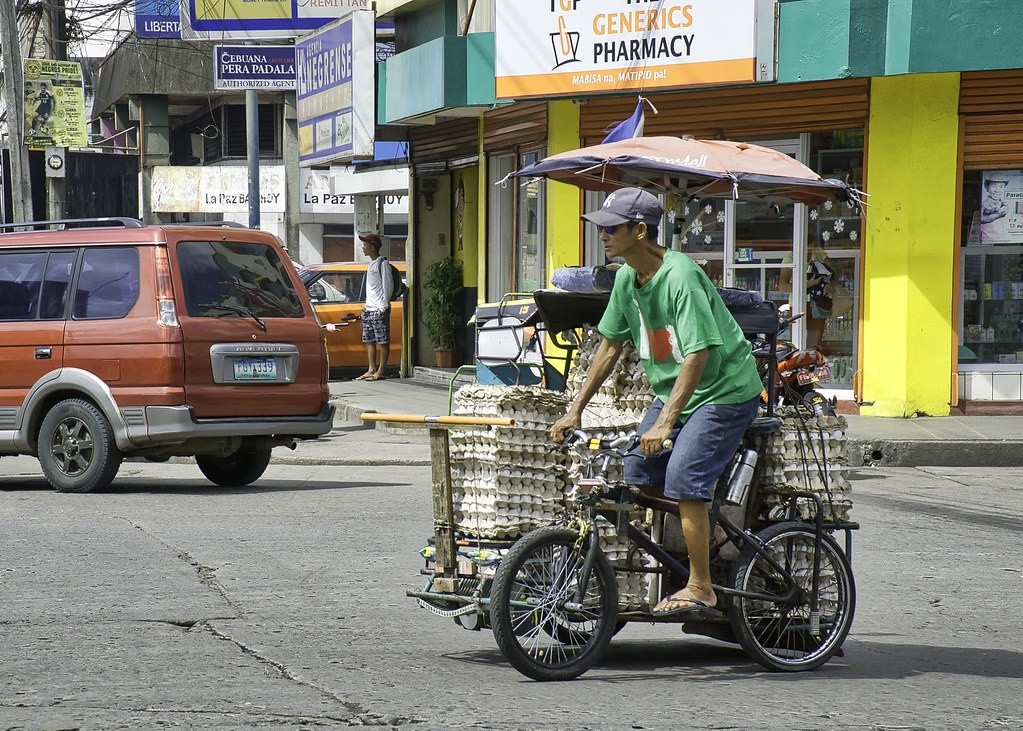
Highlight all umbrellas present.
[494,134,873,253]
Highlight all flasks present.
[725,449,758,509]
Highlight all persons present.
[550,186,763,616]
[778,232,835,348]
[24,82,55,135]
[980,173,1012,244]
[351,234,394,382]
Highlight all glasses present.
[597,222,638,234]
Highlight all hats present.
[580,186,665,226]
[359,234,382,250]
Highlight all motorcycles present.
[750,304,837,417]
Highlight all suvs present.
[0,217,338,493]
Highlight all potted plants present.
[422,256,464,368]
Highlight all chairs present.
[716,286,781,434]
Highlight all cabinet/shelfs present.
[816,148,863,247]
[958,246,1023,364]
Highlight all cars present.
[295,262,409,373]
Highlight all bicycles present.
[487,425,858,683]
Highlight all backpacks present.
[379,256,406,301]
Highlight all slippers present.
[365,376,386,381]
[652,594,723,617]
[688,536,730,558]
[356,375,369,380]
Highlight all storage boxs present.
[1011,351,1023,364]
[994,353,1016,364]
[964,281,1023,342]
